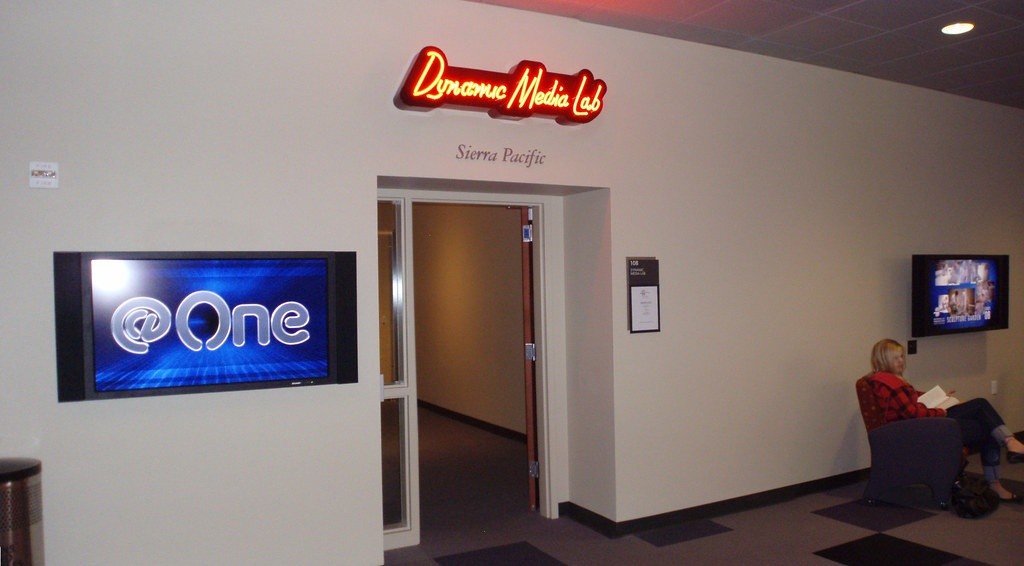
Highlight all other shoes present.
[988,486,1024,504]
[1006,450,1024,463]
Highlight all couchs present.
[856,378,964,511]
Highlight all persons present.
[936,260,964,285]
[950,290,959,314]
[938,296,947,312]
[867,339,1024,503]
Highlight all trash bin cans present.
[0,455,48,566]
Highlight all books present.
[917,385,960,410]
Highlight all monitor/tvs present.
[912,253,1010,338]
[52,251,359,405]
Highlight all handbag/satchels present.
[952,472,999,519]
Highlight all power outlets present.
[991,380,997,394]
